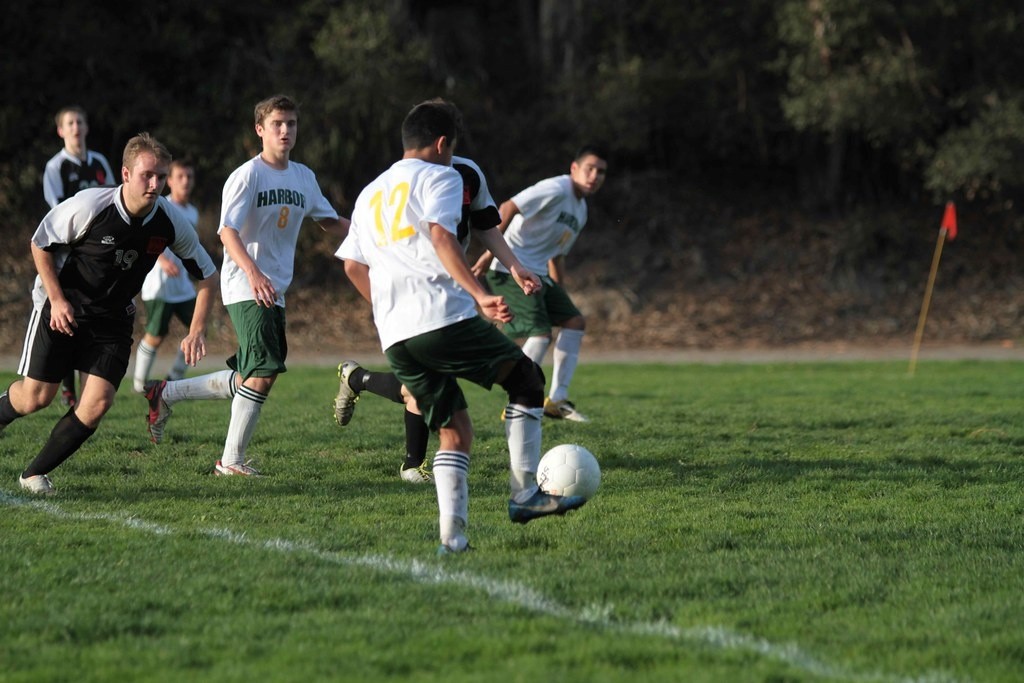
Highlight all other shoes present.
[133,376,145,393]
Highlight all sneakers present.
[214,459,259,475]
[143,379,172,445]
[437,540,474,557]
[501,408,506,421]
[19,472,55,493]
[507,486,586,523]
[543,396,589,422]
[61,389,76,408]
[333,359,360,426]
[401,459,433,483]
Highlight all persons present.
[0,93,613,494]
[336,101,589,557]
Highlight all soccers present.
[535,442,602,500]
